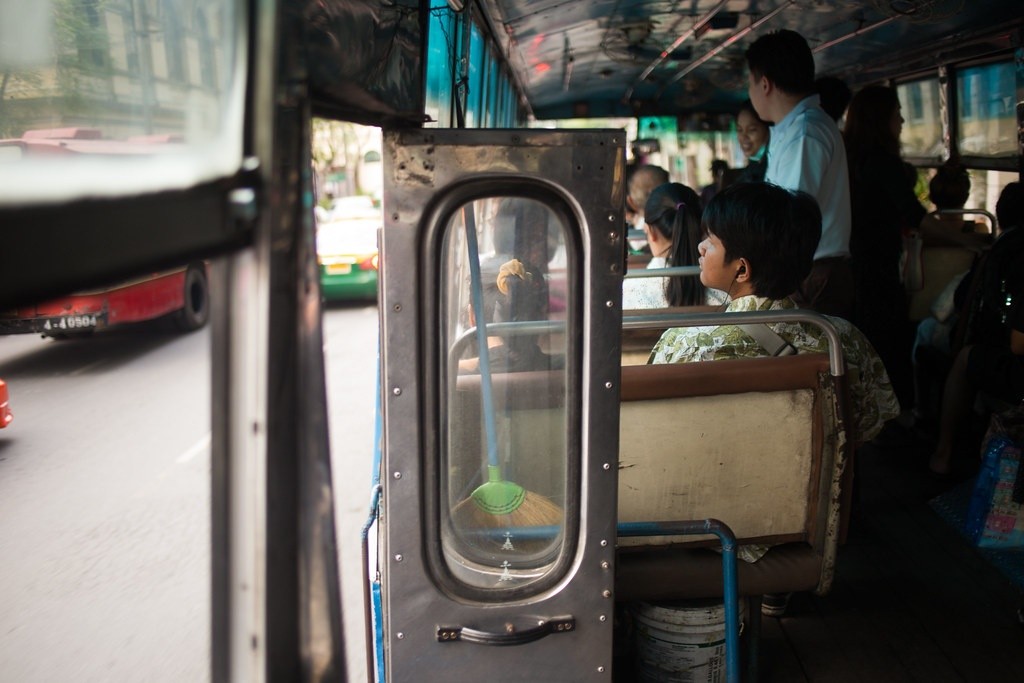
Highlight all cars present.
[1,126,380,342]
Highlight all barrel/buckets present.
[633,590,745,683]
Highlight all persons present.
[648,181,900,615]
[897,182,1024,428]
[494,197,561,273]
[626,165,669,254]
[709,161,729,182]
[746,29,856,317]
[622,183,731,310]
[926,295,1023,472]
[929,165,970,222]
[735,99,770,182]
[467,254,565,374]
[843,85,990,448]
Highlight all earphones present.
[735,265,745,277]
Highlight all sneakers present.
[761,591,792,616]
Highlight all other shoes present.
[927,449,950,475]
[872,418,920,448]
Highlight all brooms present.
[448,73,570,558]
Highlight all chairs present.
[904,207,998,325]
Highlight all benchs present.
[458,265,728,369]
[447,311,843,682]
